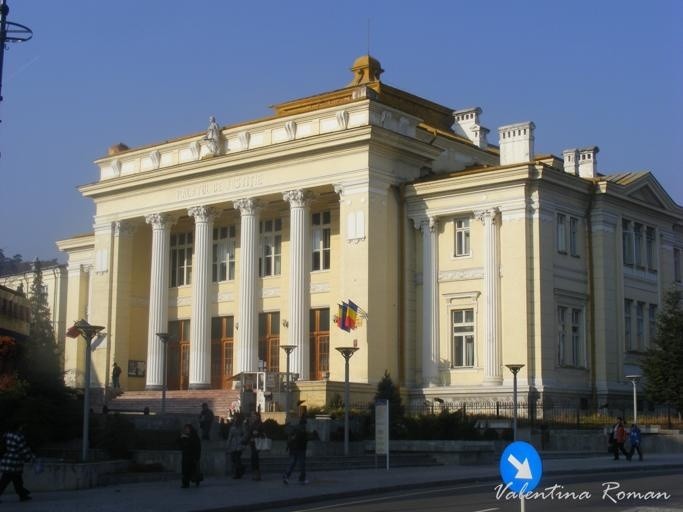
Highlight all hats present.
[200,402,208,406]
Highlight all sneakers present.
[279,473,311,486]
[231,474,261,481]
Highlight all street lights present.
[156,332,177,412]
[75,325,105,461]
[626,375,643,421]
[279,345,297,412]
[334,347,360,454]
[505,364,526,441]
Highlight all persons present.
[336,299,358,333]
[239,410,263,482]
[608,416,630,460]
[225,419,246,481]
[617,420,630,453]
[0,419,40,505]
[281,422,307,484]
[625,423,643,462]
[178,423,202,488]
[198,403,213,433]
[111,362,122,388]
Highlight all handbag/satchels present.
[253,433,272,451]
